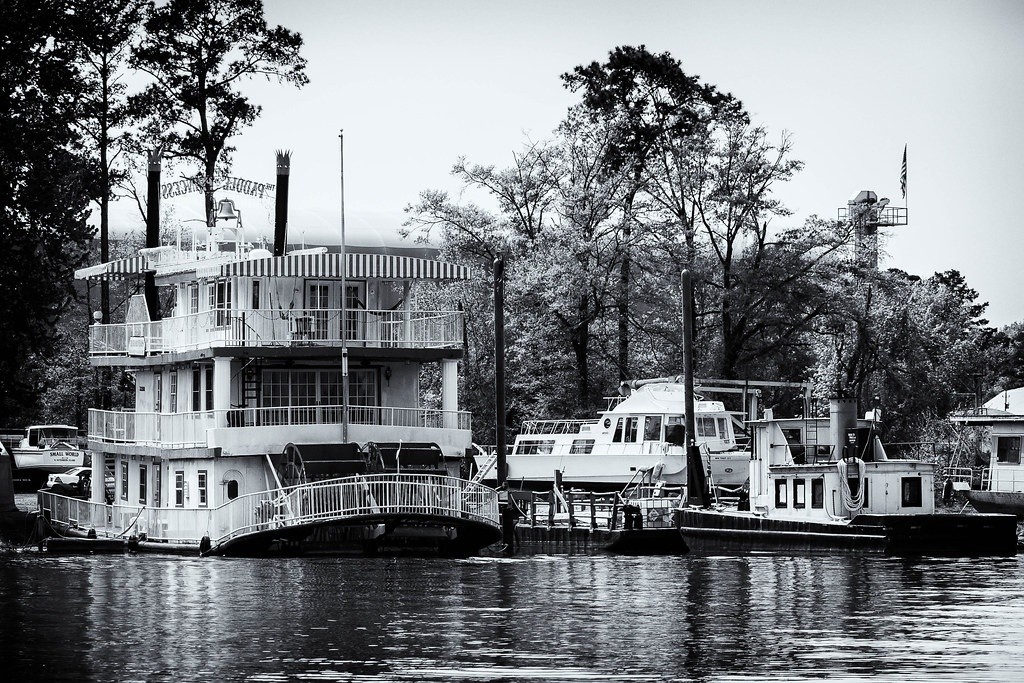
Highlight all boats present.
[11,423,89,473]
[474,379,752,507]
[669,395,1018,559]
[36,221,504,565]
[948,385,1024,518]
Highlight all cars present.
[45,466,115,493]
